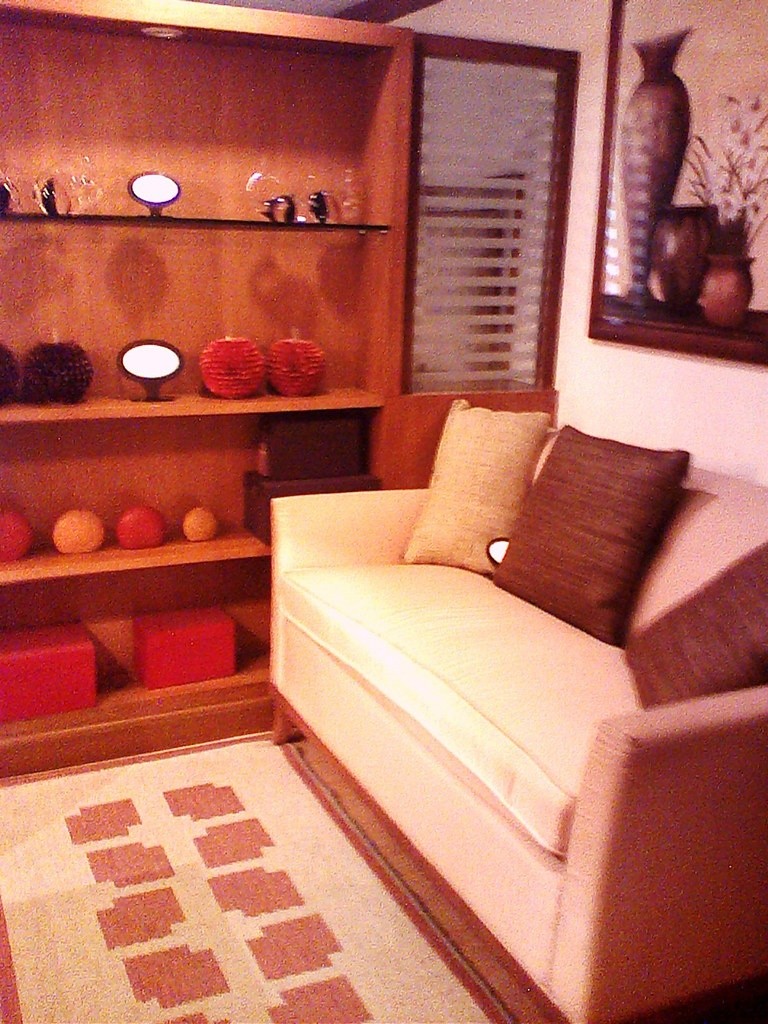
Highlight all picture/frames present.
[589,0,768,371]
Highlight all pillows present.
[628,540,768,709]
[496,423,691,650]
[405,399,548,571]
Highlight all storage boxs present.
[0,620,98,724]
[243,469,381,544]
[132,605,238,689]
[244,413,380,479]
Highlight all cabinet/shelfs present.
[0,0,416,782]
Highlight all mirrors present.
[117,340,183,402]
[129,171,181,216]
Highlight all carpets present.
[0,729,514,1024]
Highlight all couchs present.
[269,439,768,1024]
